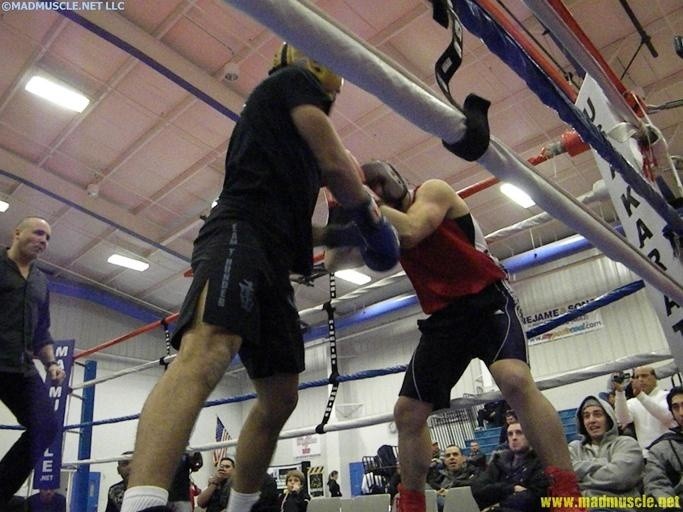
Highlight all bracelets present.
[43,362,59,370]
[212,482,219,486]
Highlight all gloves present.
[354,196,400,272]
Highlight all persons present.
[24,489,66,512]
[268,470,311,512]
[105,451,133,512]
[189,478,201,512]
[197,458,235,512]
[324,157,585,512]
[0,216,66,512]
[118,37,401,512]
[362,365,682,512]
[327,471,343,497]
[165,441,202,512]
[222,466,278,512]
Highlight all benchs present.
[461,408,579,456]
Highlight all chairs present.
[304,486,479,511]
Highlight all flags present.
[214,418,231,466]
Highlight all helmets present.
[272,41,345,99]
[359,161,411,212]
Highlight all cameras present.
[611,372,630,383]
